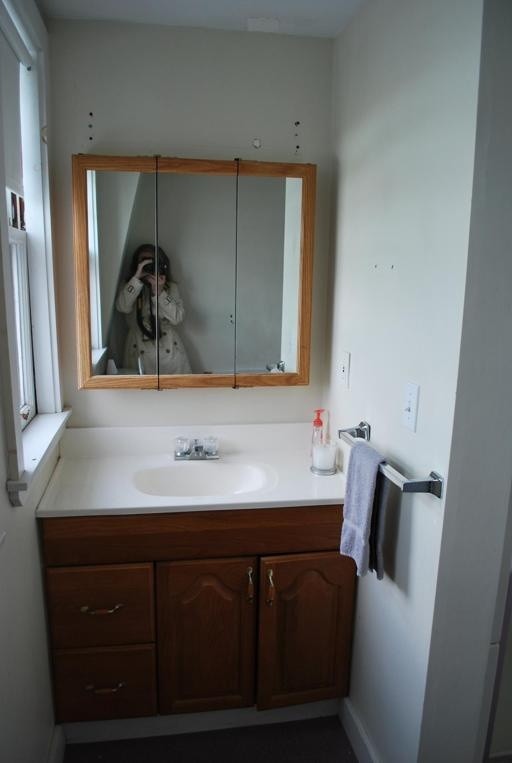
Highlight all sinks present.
[132,463,275,498]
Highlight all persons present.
[116,244,193,374]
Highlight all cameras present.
[143,257,169,275]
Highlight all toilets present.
[106,354,146,376]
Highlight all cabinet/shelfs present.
[157,546,360,716]
[43,559,158,719]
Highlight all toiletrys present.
[311,407,328,458]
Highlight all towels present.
[341,439,389,582]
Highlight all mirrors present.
[70,151,311,387]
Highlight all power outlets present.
[336,351,350,387]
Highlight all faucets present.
[185,438,207,460]
